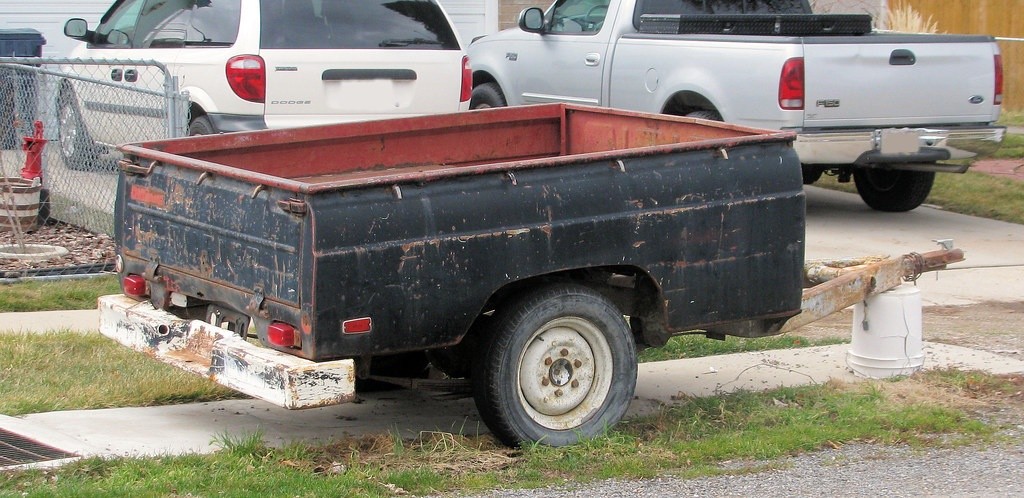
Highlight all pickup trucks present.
[465,1,1007,213]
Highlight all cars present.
[56,0,473,180]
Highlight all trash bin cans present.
[0,27,46,150]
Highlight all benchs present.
[277,13,391,47]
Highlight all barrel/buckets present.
[845,283,926,380]
[0,177,42,235]
